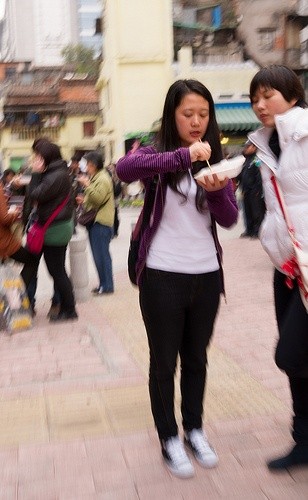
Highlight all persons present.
[239,138,267,239]
[0,137,115,324]
[247,64,308,473]
[116,79,239,479]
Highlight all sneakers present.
[183,429,218,468]
[160,434,196,478]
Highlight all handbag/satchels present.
[26,222,44,255]
[0,259,34,336]
[128,239,140,285]
[77,208,97,226]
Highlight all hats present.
[241,140,253,147]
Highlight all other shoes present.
[268,443,308,471]
[92,288,114,296]
[47,310,78,323]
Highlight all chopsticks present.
[199,139,212,172]
